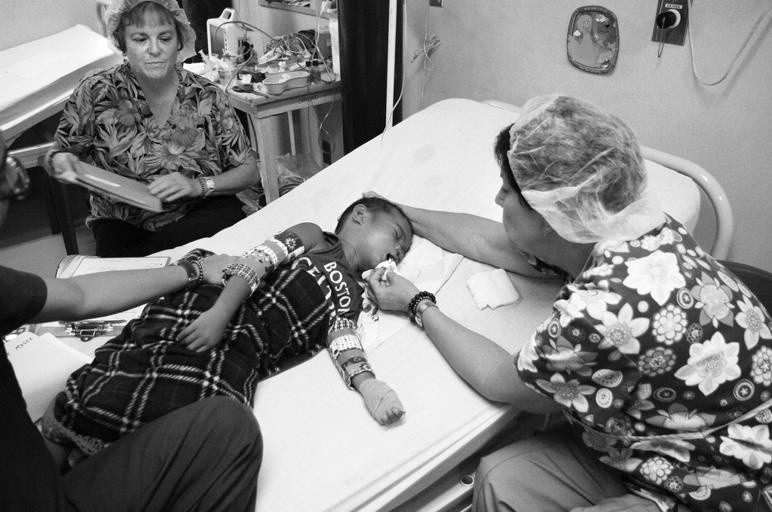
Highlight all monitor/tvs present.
[206,7,264,65]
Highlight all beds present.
[3,97,733,512]
[0,24,126,255]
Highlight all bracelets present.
[177,257,205,287]
[201,176,216,199]
[407,290,439,330]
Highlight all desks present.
[198,71,343,212]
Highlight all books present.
[67,158,164,214]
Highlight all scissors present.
[233,84,269,99]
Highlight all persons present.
[40,194,416,468]
[0,128,234,511]
[42,1,266,258]
[365,90,772,512]
